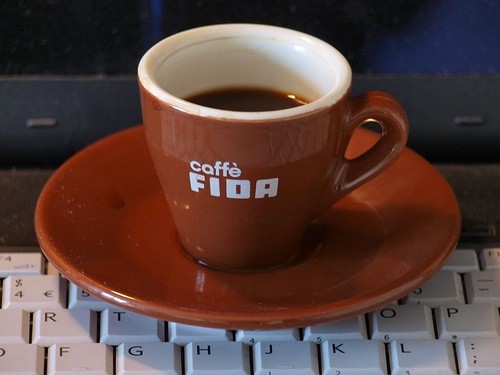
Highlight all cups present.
[137,23,409,273]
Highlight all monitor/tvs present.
[0,0,499,163]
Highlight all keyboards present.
[1,248,500,375]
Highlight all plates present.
[33,123,461,329]
